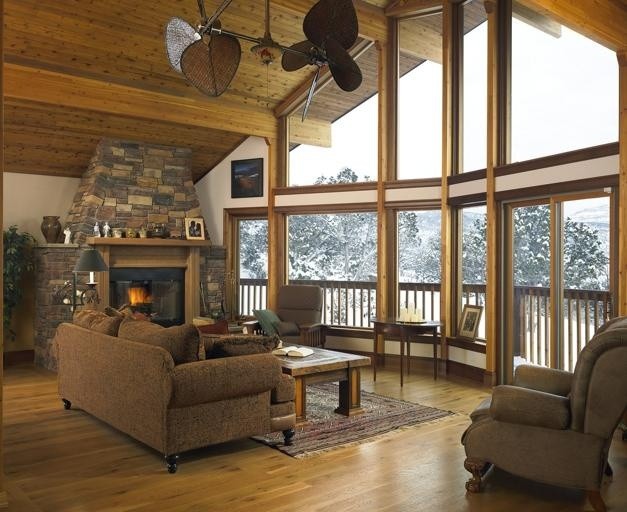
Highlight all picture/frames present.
[231,158,263,198]
[185,218,204,241]
[456,305,483,342]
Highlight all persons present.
[189,220,201,237]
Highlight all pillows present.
[272,321,298,336]
[73,303,276,365]
[252,309,283,335]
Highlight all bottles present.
[103,222,110,237]
[94,222,101,237]
[400,302,422,322]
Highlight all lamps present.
[72,251,108,323]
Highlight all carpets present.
[250,377,454,460]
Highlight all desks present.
[369,312,444,386]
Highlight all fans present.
[164,0,363,123]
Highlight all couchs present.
[54,304,296,474]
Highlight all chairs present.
[243,284,327,347]
[461,317,627,512]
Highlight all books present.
[271,346,315,357]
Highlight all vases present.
[41,216,61,244]
